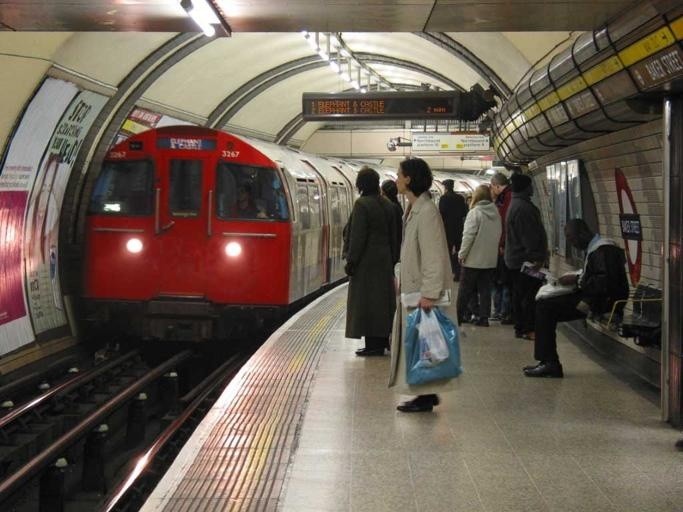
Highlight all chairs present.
[586,282,663,363]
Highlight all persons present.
[436,167,550,346]
[224,180,270,219]
[339,167,403,357]
[393,157,453,414]
[381,178,404,266]
[520,216,632,377]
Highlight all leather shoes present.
[355,346,385,356]
[492,308,533,339]
[396,398,433,412]
[522,362,563,378]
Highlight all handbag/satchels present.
[534,268,584,301]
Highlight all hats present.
[509,172,532,193]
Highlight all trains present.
[75,123,495,353]
